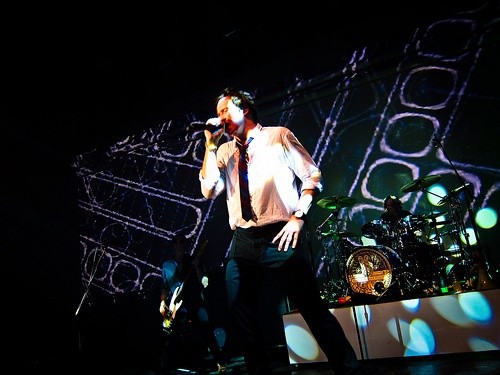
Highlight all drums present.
[394,215,426,233]
[375,231,419,254]
[344,246,405,300]
[362,220,390,237]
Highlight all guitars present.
[163,239,210,334]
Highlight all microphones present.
[435,138,441,148]
[190,121,224,133]
[375,282,386,292]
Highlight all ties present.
[235,137,254,222]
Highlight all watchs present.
[292,210,306,221]
[205,142,217,151]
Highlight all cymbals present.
[438,183,470,204]
[317,196,357,209]
[323,232,362,237]
[400,175,441,194]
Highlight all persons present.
[197,88,361,375]
[380,195,414,227]
[158,233,226,375]
